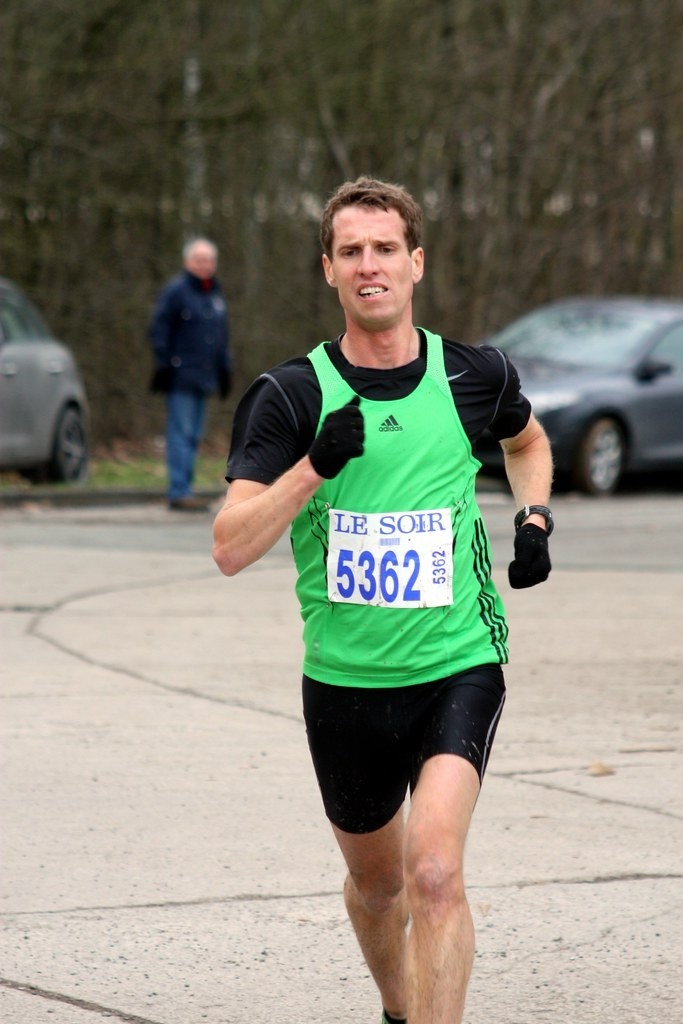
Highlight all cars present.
[1,280,91,489]
[463,298,682,499]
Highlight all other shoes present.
[170,496,207,509]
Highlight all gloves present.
[148,368,171,396]
[308,395,364,479]
[217,369,233,401]
[508,523,551,589]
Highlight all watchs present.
[515,505,554,537]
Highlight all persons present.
[147,237,231,514]
[213,178,554,1023]
